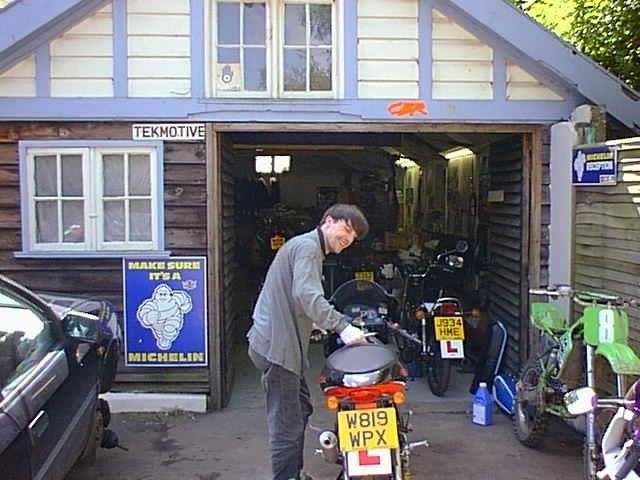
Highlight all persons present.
[245,204,373,480]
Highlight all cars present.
[0,271,125,479]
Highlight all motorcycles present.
[386,238,481,396]
[309,277,432,480]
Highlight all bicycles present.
[510,283,639,480]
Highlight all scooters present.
[563,382,639,479]
